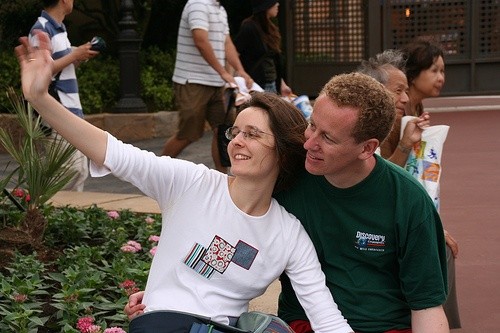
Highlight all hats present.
[252,0,278,15]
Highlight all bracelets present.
[399,141,413,154]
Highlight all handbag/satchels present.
[217,124,236,167]
[20,85,61,116]
[400,116,450,215]
[287,94,313,119]
[237,311,295,333]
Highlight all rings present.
[29,58,36,61]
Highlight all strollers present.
[223,77,313,126]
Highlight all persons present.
[161,0,295,174]
[15,29,355,333]
[28,0,100,192]
[362,38,461,330]
[125,74,450,333]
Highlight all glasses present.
[225,127,274,141]
